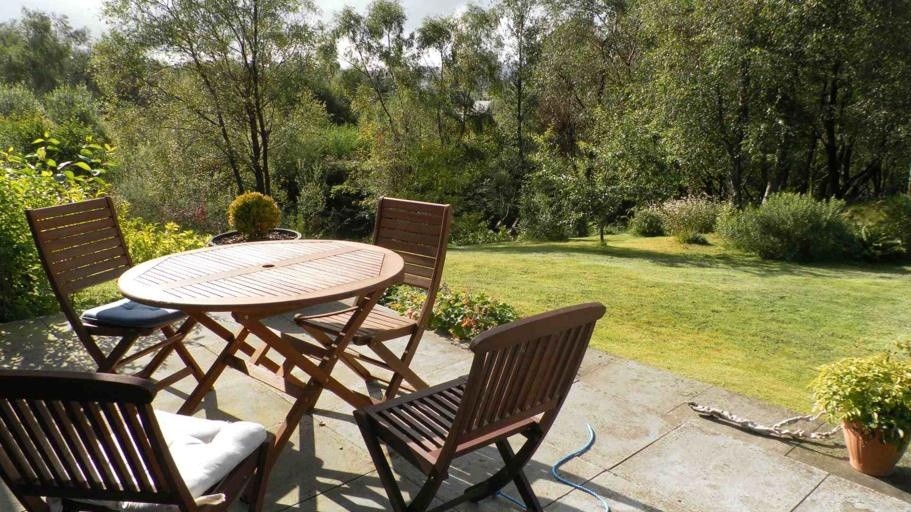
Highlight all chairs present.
[0,369,274,512]
[25,197,214,414]
[354,301,605,512]
[295,198,451,401]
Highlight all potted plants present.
[208,192,303,247]
[807,338,911,477]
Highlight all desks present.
[119,239,404,501]
